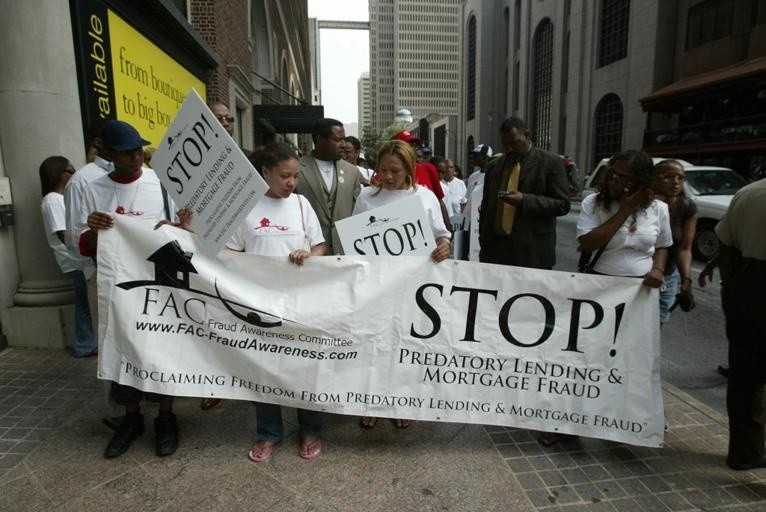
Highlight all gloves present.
[678,277,696,313]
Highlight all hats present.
[420,147,434,154]
[468,143,493,159]
[100,120,151,153]
[390,129,421,146]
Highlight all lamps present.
[0,176,15,227]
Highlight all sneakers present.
[200,398,220,410]
[100,412,127,430]
[538,431,562,447]
[718,365,730,376]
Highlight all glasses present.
[658,174,688,184]
[607,166,632,185]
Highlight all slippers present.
[248,439,274,462]
[298,433,322,460]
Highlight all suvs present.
[580,156,748,263]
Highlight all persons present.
[38,103,766,470]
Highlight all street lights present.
[394,107,415,131]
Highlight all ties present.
[501,159,521,236]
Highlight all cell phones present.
[498,190,511,195]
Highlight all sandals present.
[393,419,412,428]
[359,416,377,430]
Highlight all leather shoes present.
[104,406,145,463]
[152,410,179,456]
